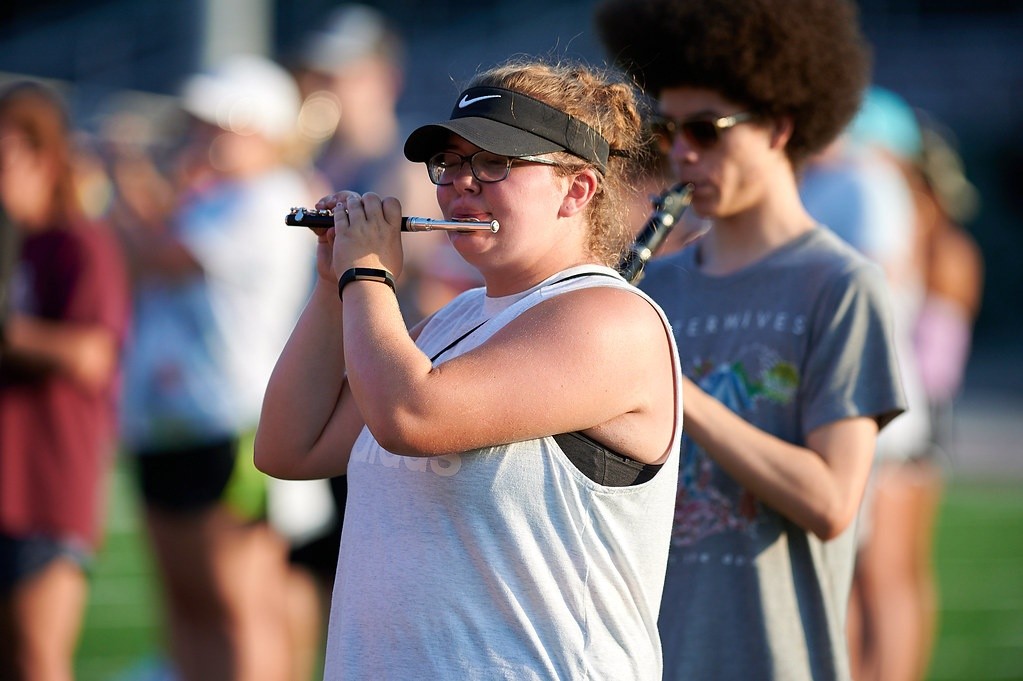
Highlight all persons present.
[598,0,911,681]
[0,82,131,679]
[253,65,684,681]
[108,8,406,680]
[794,87,982,680]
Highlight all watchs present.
[338,267,399,302]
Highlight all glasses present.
[644,107,762,151]
[424,152,602,194]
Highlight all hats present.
[177,55,301,140]
[404,87,630,178]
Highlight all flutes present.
[613,180,694,282]
[284,207,499,233]
